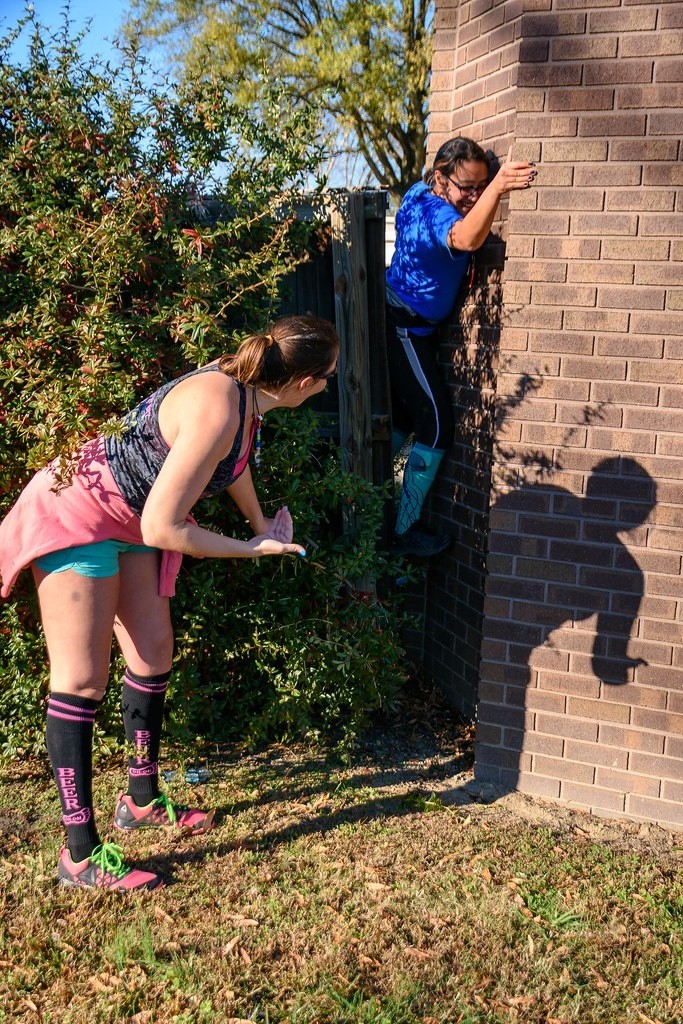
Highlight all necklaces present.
[251,388,264,468]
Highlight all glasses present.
[309,366,337,379]
[444,173,487,196]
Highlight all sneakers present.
[113,790,215,834]
[57,842,163,892]
[389,518,450,558]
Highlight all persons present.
[384,136,537,556]
[0,316,339,894]
